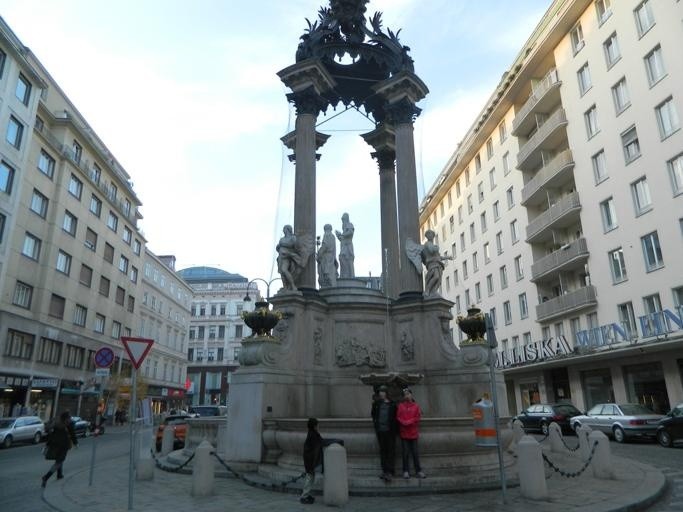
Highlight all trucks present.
[187,405,227,416]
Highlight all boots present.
[57,466,64,478]
[42,471,52,487]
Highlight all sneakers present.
[301,495,314,504]
[381,473,393,481]
[415,472,426,478]
[403,471,409,478]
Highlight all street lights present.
[243,278,282,336]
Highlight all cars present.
[160,409,186,416]
[43,416,91,438]
[511,402,583,435]
[569,403,667,443]
[656,401,682,447]
[156,413,200,452]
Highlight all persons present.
[39,407,78,489]
[275,224,304,297]
[369,385,398,482]
[419,229,453,298]
[333,213,356,280]
[119,408,125,426]
[395,387,427,480]
[298,416,345,505]
[113,407,120,426]
[314,223,338,291]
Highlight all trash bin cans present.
[472,393,497,446]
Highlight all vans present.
[214,397,216,404]
[0,415,48,449]
